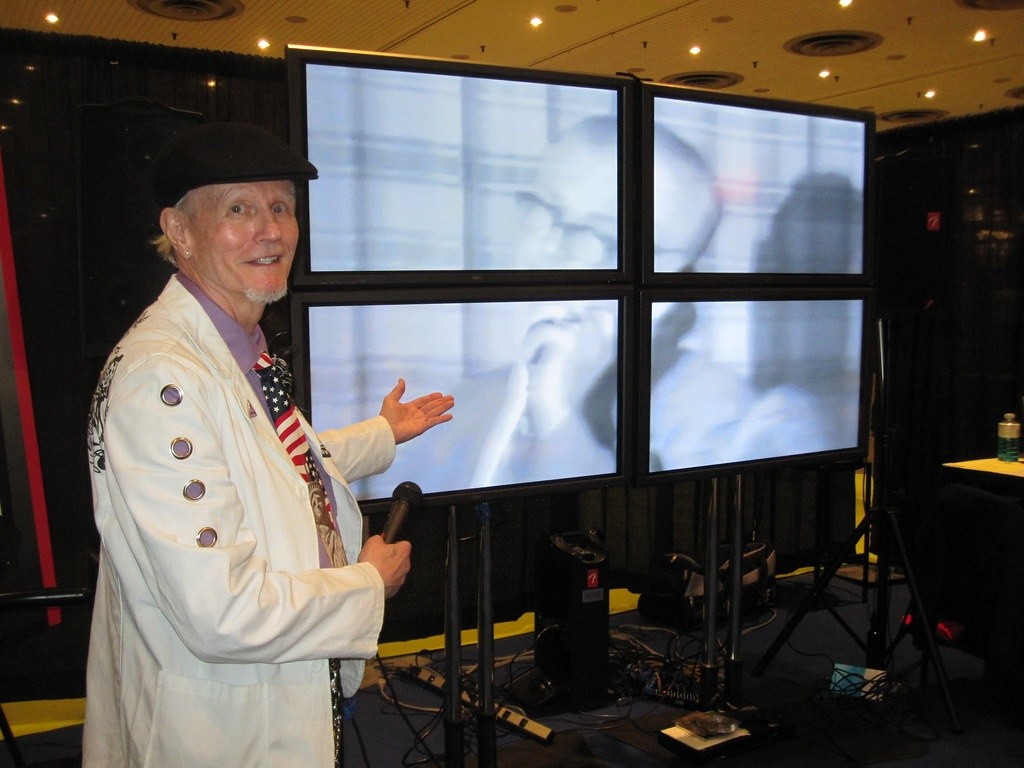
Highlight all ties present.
[253,352,349,570]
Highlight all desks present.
[941,452,1024,512]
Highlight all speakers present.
[69,92,206,359]
[874,152,973,314]
[531,526,615,711]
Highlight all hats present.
[167,122,318,207]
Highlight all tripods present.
[745,305,965,734]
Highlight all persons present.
[465,113,780,487]
[81,117,455,768]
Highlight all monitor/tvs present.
[632,285,875,485]
[284,43,638,288]
[289,286,637,522]
[633,81,877,286]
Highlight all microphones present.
[382,481,425,546]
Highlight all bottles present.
[998,414,1021,462]
